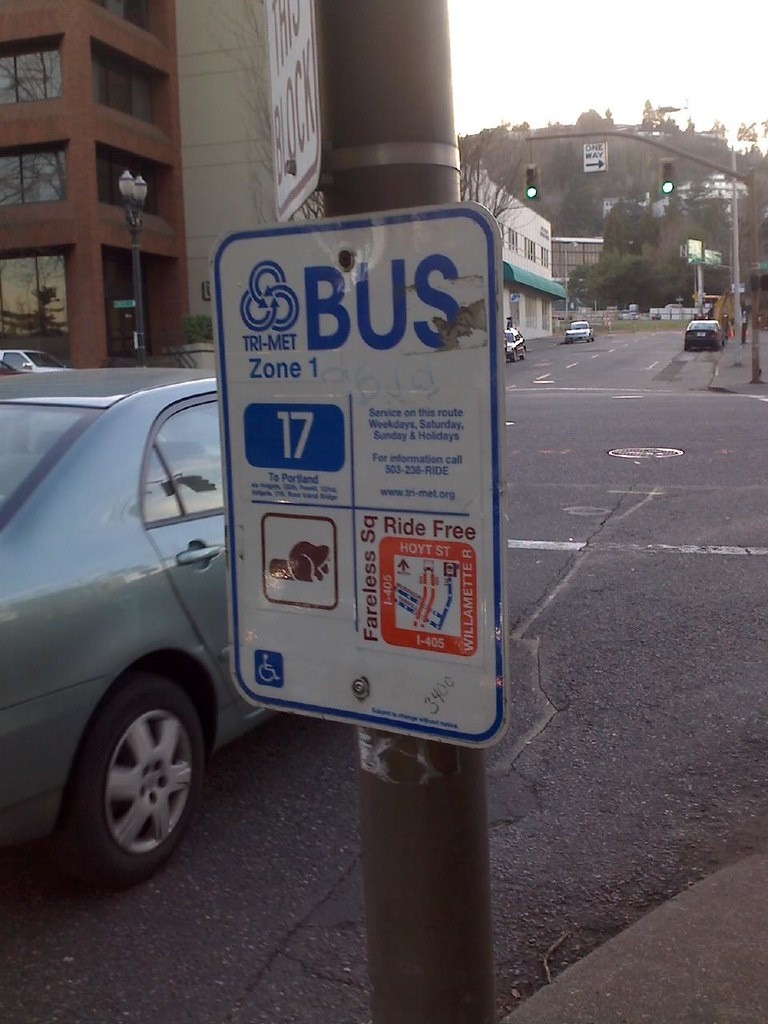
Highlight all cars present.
[0,349,80,379]
[565,319,594,344]
[0,370,305,892]
[504,327,527,363]
[618,309,639,320]
[683,319,726,352]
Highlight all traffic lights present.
[524,163,542,202]
[659,157,678,194]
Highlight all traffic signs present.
[583,141,608,173]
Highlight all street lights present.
[117,167,150,371]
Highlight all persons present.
[694,314,706,320]
[507,317,513,329]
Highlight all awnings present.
[503,261,567,299]
